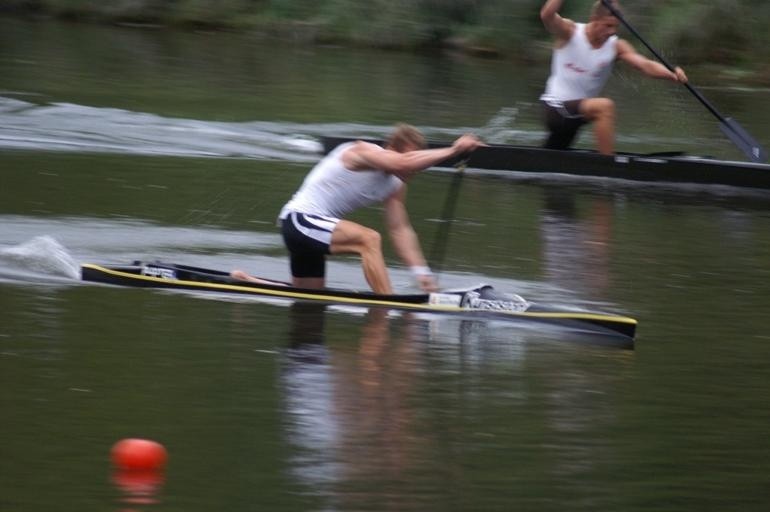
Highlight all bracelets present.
[409,265,434,276]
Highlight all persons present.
[226,119,493,292]
[536,0,689,153]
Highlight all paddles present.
[600,0,763,164]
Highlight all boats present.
[80,252,639,354]
[313,127,769,206]
